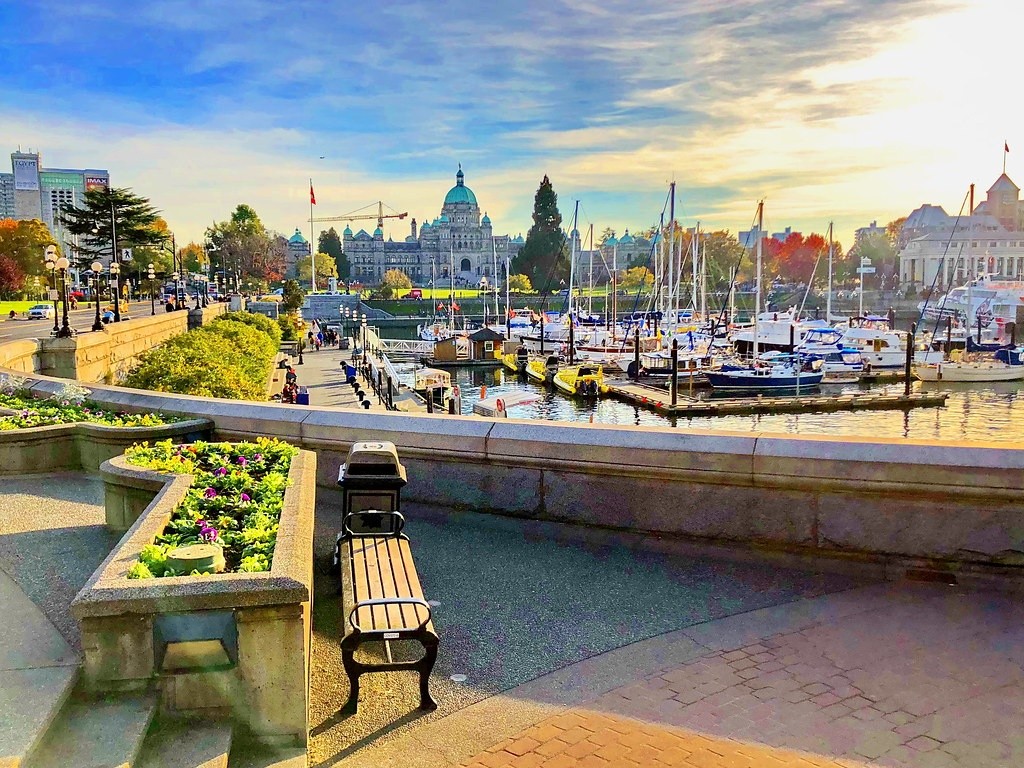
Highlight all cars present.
[107,299,129,313]
[160,292,233,305]
[27,304,55,320]
[101,311,116,323]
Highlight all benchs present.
[335,510,439,714]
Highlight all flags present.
[311,185,316,204]
[531,309,548,325]
[1005,144,1009,152]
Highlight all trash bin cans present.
[346,365,356,383]
[337,440,407,537]
[296,388,310,406]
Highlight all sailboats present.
[381,178,1024,396]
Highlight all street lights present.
[159,233,182,310]
[55,257,76,338]
[193,273,242,309]
[171,271,182,310]
[90,261,106,332]
[109,262,122,322]
[91,201,122,321]
[45,244,59,334]
[148,263,156,315]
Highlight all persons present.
[279,359,290,369]
[308,330,338,352]
[312,320,315,329]
[124,250,130,259]
[284,369,298,404]
[103,308,115,323]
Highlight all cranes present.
[308,201,407,227]
